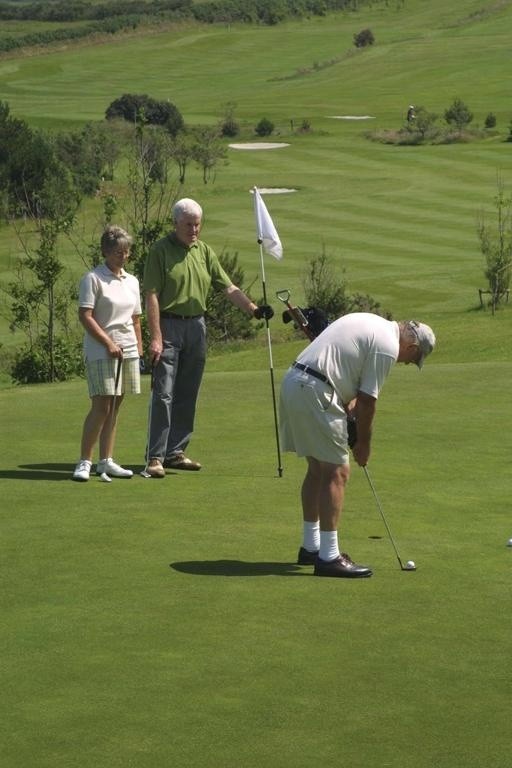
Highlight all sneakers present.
[96,458,133,479]
[144,458,166,478]
[73,459,93,482]
[165,455,201,471]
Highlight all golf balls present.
[506,538,512,546]
[404,561,414,568]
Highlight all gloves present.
[347,424,357,449]
[253,304,274,321]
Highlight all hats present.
[408,318,435,369]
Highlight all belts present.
[291,360,333,387]
[160,310,207,322]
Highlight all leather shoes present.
[313,554,372,578]
[297,547,354,566]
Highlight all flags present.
[252,181,284,260]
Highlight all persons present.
[279,312,435,578]
[72,225,142,478]
[142,198,274,478]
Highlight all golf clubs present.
[362,465,418,572]
[141,355,152,478]
[101,347,111,481]
